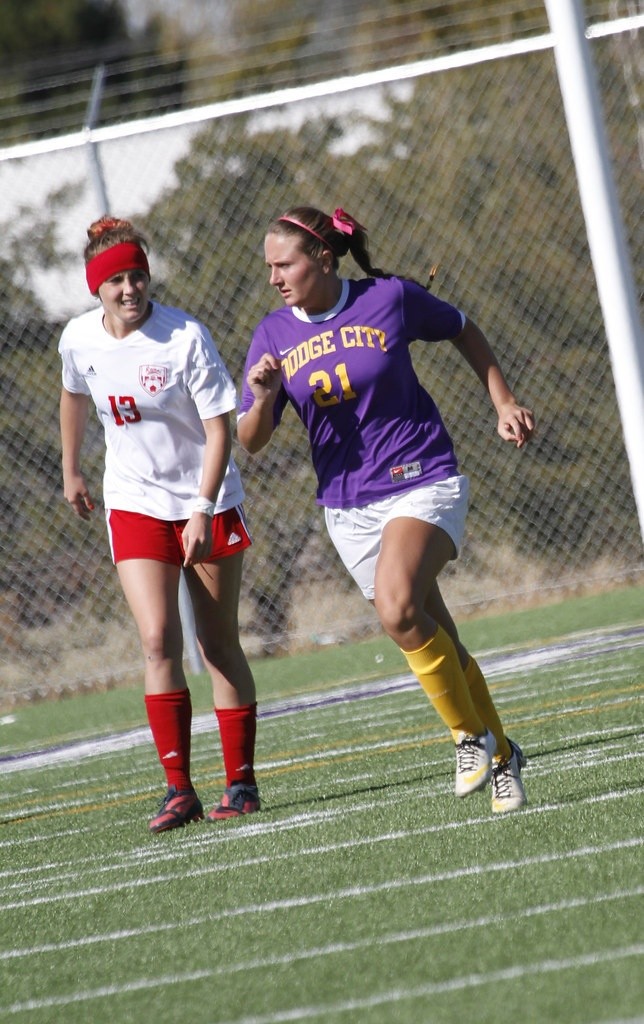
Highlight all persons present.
[234,203,537,816]
[58,215,264,832]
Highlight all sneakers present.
[454,728,497,798]
[491,737,529,814]
[205,782,260,821]
[149,786,205,833]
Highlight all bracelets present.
[194,497,216,518]
